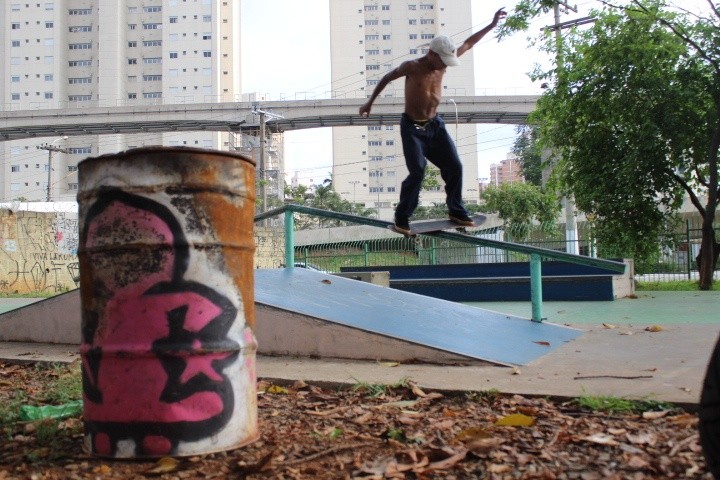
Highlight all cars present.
[294,262,335,273]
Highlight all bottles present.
[19,400,82,423]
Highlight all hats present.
[429,35,457,65]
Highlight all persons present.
[359,6,508,235]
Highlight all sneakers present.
[449,211,474,225]
[395,216,412,235]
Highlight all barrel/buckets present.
[76,146,261,458]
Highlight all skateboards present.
[386,214,487,244]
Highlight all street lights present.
[348,180,361,208]
[46,136,69,202]
[448,99,458,151]
